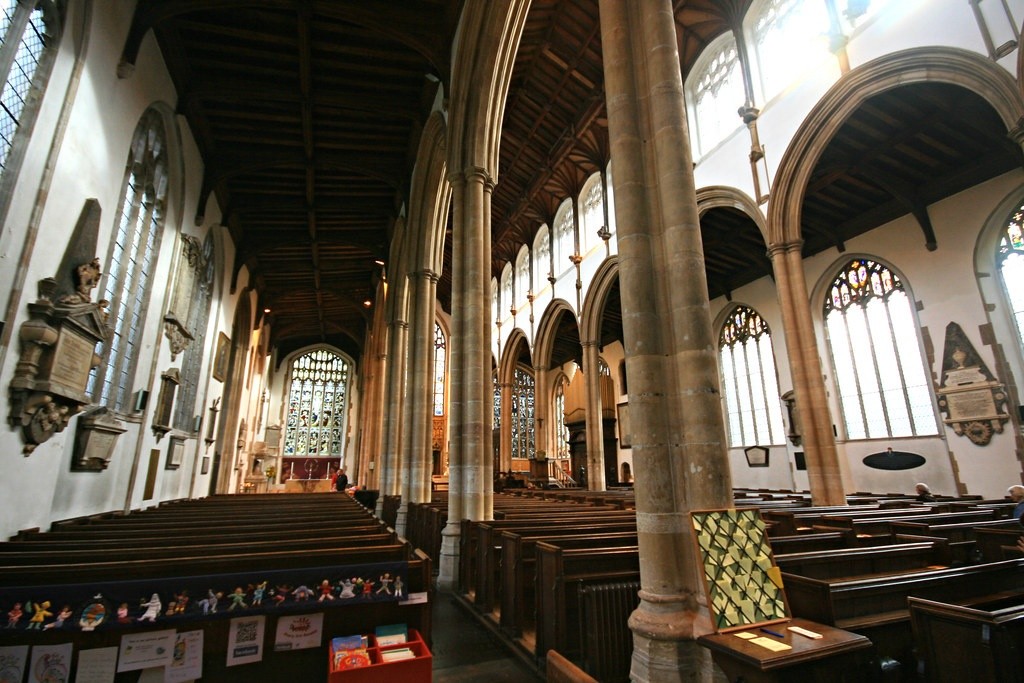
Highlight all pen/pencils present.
[759,627,784,638]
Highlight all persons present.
[339,578,356,599]
[292,583,314,602]
[358,576,376,599]
[916,483,937,502]
[335,470,348,491]
[43,604,73,630]
[317,580,336,604]
[137,593,162,622]
[376,573,394,596]
[1016,513,1024,553]
[250,581,268,607]
[26,600,54,629]
[174,591,189,612]
[5,601,23,629]
[272,583,292,607]
[198,589,224,613]
[282,466,299,482]
[393,576,404,598]
[117,602,131,623]
[1008,485,1024,518]
[227,587,248,612]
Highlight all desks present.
[696,618,873,683]
[285,479,332,493]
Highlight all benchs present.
[0,481,1024,683]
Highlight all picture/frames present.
[689,507,792,634]
[617,402,631,449]
[213,332,231,382]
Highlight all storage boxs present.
[329,630,433,683]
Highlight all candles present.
[327,462,330,479]
[290,462,294,479]
